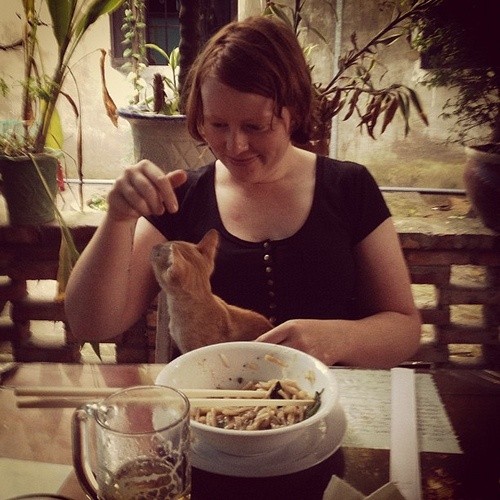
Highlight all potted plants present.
[0,0,123,361]
[115,44,217,172]
[406,0,500,231]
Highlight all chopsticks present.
[14,386,316,408]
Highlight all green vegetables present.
[264,380,325,422]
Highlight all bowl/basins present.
[154,341,339,457]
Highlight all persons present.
[70,15,421,367]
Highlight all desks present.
[1,363,500,499]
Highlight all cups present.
[71,383,191,500]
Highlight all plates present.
[151,400,349,477]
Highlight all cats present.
[149,229,275,354]
